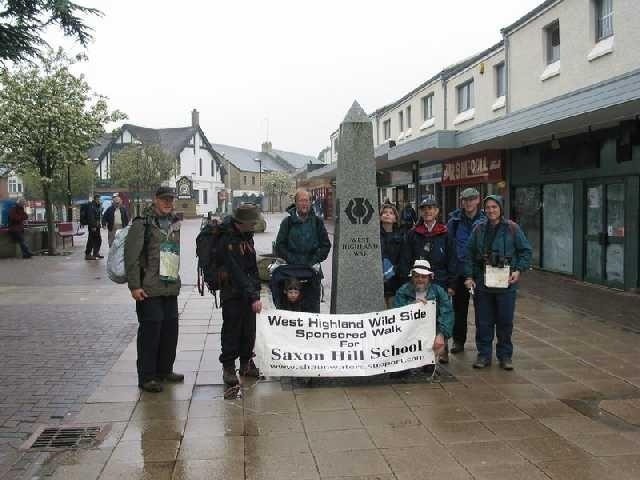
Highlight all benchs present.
[58,224,77,249]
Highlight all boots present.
[238,358,259,377]
[222,362,240,386]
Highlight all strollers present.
[271,263,320,384]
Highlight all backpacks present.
[79,205,88,226]
[107,218,133,284]
[196,217,223,307]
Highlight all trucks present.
[232,189,266,233]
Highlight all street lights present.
[271,182,288,214]
[68,157,99,222]
[133,140,142,217]
[253,158,261,195]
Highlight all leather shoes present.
[156,372,184,383]
[499,358,514,370]
[450,342,464,353]
[84,255,96,260]
[438,349,448,363]
[140,380,161,392]
[93,253,104,258]
[472,355,492,368]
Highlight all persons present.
[283,277,304,305]
[122,185,184,393]
[85,193,104,260]
[276,189,332,271]
[378,188,532,378]
[8,199,37,258]
[218,202,268,387]
[101,192,128,248]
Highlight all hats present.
[462,187,480,200]
[418,198,438,209]
[155,186,179,199]
[232,202,264,225]
[407,259,435,280]
